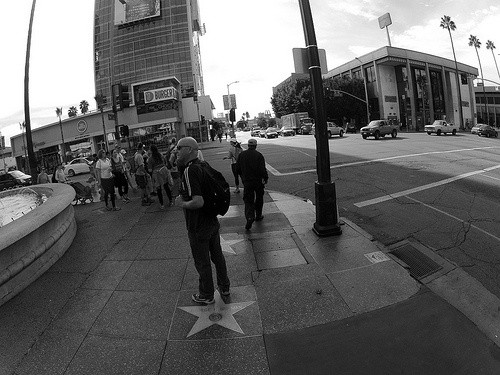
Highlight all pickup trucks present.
[360,120,400,140]
[424,120,459,136]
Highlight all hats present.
[248,138,257,145]
[230,138,237,142]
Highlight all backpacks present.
[196,164,230,215]
[165,153,177,169]
[232,146,243,160]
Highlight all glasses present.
[177,145,190,150]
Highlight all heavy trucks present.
[281,112,313,135]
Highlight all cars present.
[226,124,281,139]
[471,124,498,138]
[0,169,32,190]
[60,157,94,177]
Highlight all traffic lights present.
[326,87,330,96]
[111,83,130,112]
[120,125,129,138]
[461,73,468,85]
[193,91,198,102]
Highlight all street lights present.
[355,57,370,124]
[227,81,239,124]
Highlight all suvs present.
[345,122,357,134]
[311,121,344,139]
[280,126,295,136]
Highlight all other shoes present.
[142,200,151,206]
[160,204,164,209]
[112,207,120,211]
[256,215,264,222]
[234,189,240,193]
[245,217,255,229]
[167,203,173,206]
[105,206,111,211]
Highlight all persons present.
[223,138,242,194]
[50,162,69,184]
[86,137,181,211]
[36,168,51,184]
[235,139,269,230]
[173,137,231,304]
[197,150,204,162]
[209,129,228,143]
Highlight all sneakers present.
[219,287,230,296]
[191,293,215,304]
